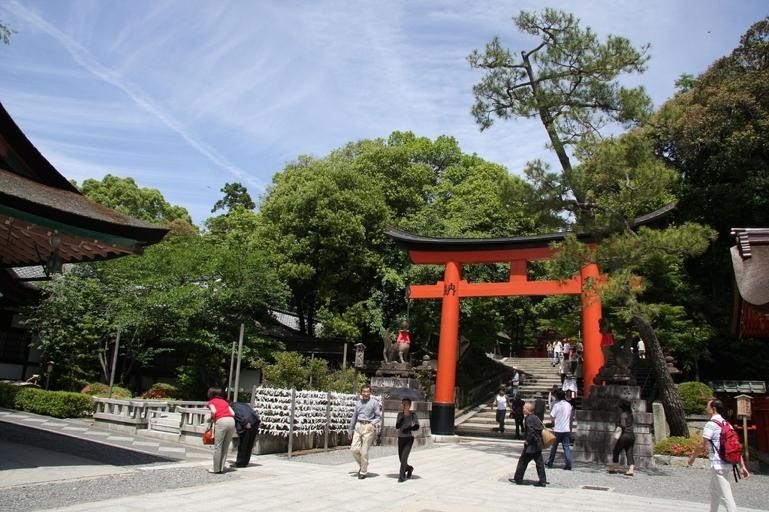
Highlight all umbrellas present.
[388,388,424,414]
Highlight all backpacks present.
[710,419,743,464]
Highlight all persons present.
[393,398,419,483]
[507,402,547,488]
[511,369,519,398]
[543,335,584,472]
[491,386,512,429]
[396,321,412,363]
[608,399,635,477]
[226,399,261,469]
[347,384,382,479]
[509,399,515,419]
[531,391,547,424]
[206,386,235,475]
[510,391,526,439]
[687,398,750,512]
[637,338,646,359]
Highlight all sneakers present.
[608,466,635,476]
[398,465,413,483]
[545,459,572,470]
[509,478,546,487]
[358,468,367,479]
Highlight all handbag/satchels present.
[202,419,215,445]
[540,428,557,449]
